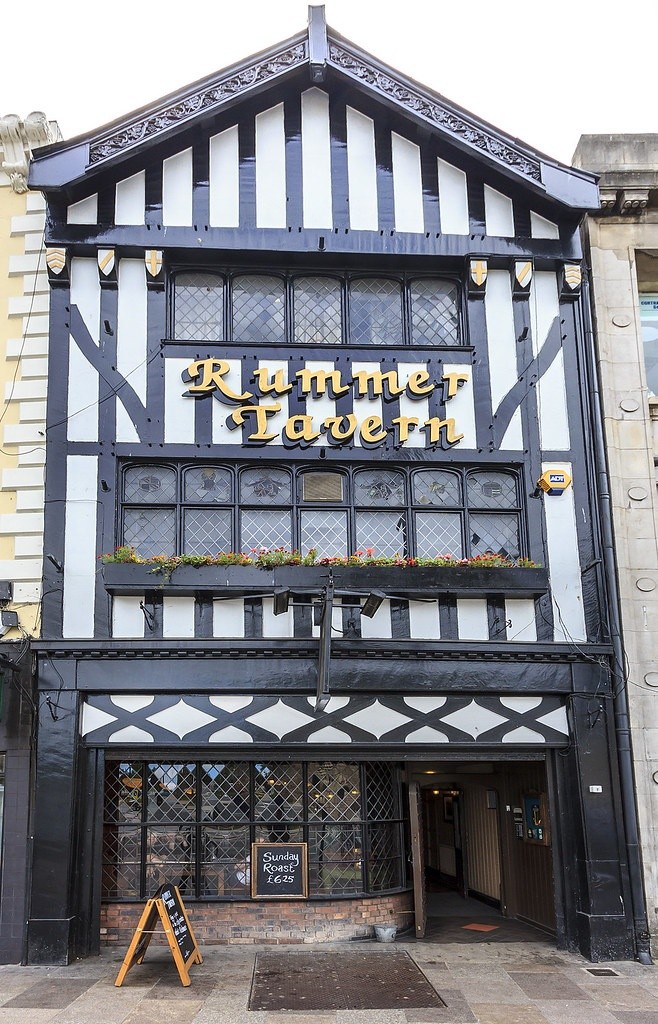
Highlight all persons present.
[146,830,168,886]
[223,826,269,889]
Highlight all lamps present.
[274,572,387,710]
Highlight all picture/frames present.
[443,794,454,824]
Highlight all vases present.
[103,565,549,587]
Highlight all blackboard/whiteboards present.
[124,884,199,973]
[251,842,309,899]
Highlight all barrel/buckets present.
[374,923,398,944]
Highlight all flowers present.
[103,545,543,581]
